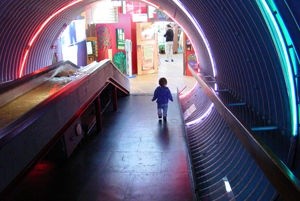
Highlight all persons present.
[151,77,173,121]
[162,24,174,62]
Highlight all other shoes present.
[159,116,166,120]
[165,59,173,62]
